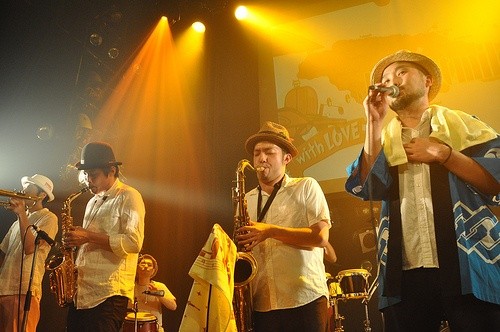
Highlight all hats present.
[137,254,158,279]
[245,121,298,159]
[370,49,442,104]
[75,142,122,170]
[20,173,55,202]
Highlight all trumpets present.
[0,189,41,209]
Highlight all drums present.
[123,312,158,332]
[338,268,370,299]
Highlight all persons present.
[126,255,179,332]
[343,49,500,332]
[233,120,334,332]
[317,241,343,332]
[0,173,59,332]
[69,140,146,332]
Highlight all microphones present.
[370,85,399,97]
[33,225,58,247]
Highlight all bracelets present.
[437,142,453,167]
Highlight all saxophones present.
[46,186,90,308]
[231,159,265,332]
